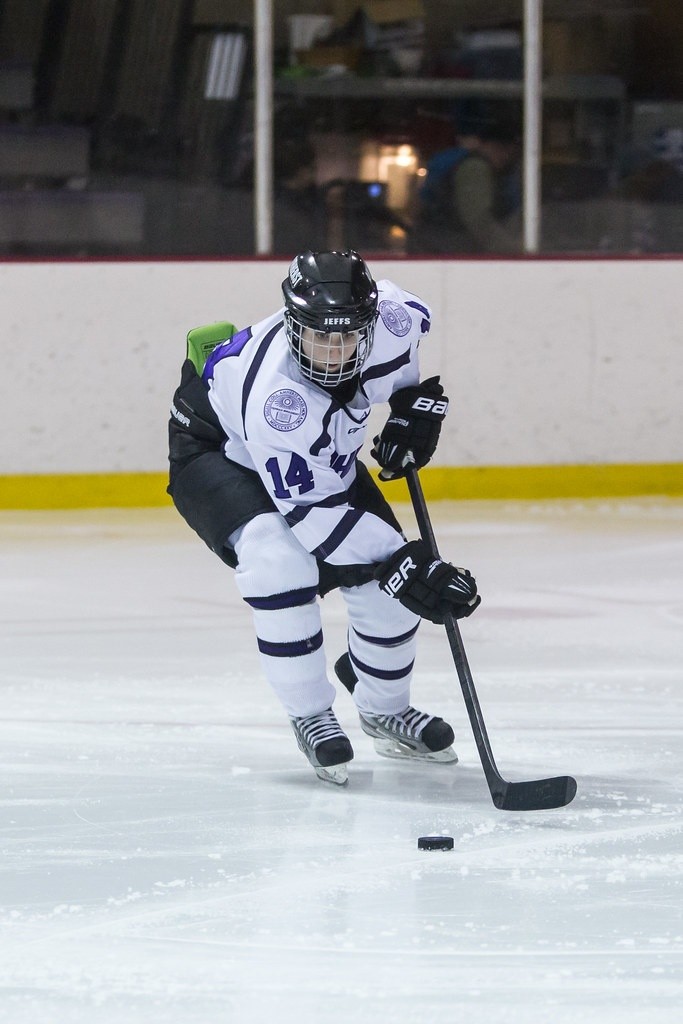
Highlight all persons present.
[166,248,482,786]
[396,121,513,251]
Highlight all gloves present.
[373,538,482,624]
[370,375,449,482]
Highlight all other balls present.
[417,837,454,851]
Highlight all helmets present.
[282,248,379,387]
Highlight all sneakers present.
[288,705,354,785]
[335,652,458,764]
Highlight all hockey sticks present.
[401,450,577,811]
